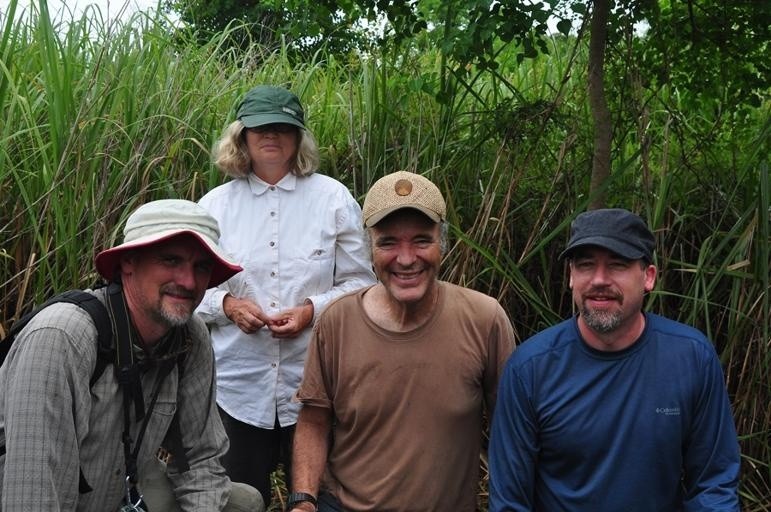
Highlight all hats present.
[557,208,656,264]
[235,85,307,132]
[361,171,446,230]
[94,199,243,291]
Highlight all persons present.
[487,207,743,511]
[0,198,266,512]
[193,87,378,511]
[292,169,516,512]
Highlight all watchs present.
[286,492,317,508]
